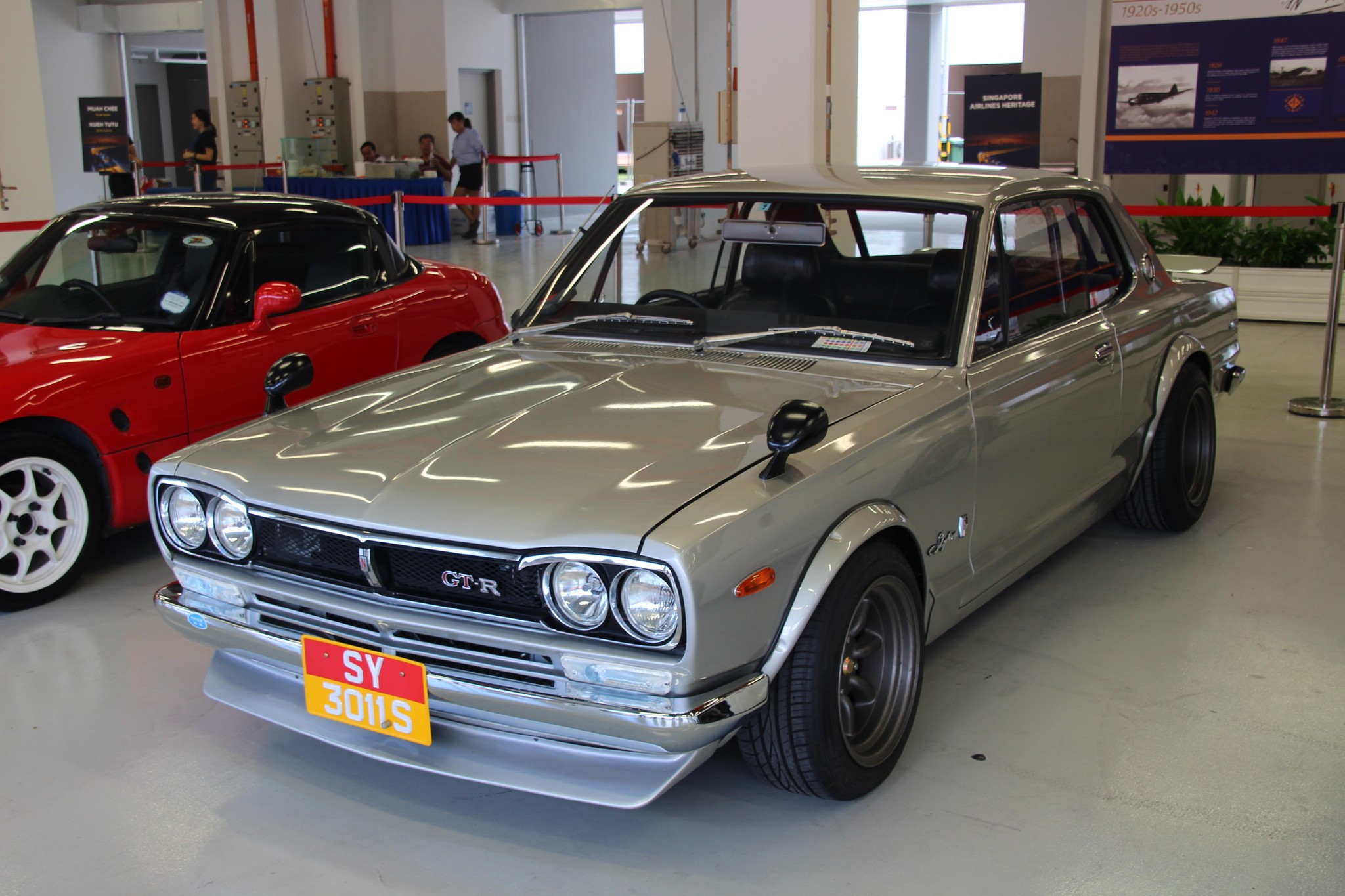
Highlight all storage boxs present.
[281,138,332,178]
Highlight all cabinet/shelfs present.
[262,176,451,245]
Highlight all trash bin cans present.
[940,137,964,163]
[493,191,521,236]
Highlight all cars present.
[0,190,509,614]
[144,160,1246,808]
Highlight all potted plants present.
[1137,184,1345,324]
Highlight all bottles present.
[679,102,686,122]
[287,161,297,177]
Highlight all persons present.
[183,109,222,192]
[418,133,453,183]
[98,129,144,199]
[359,141,383,162]
[448,112,488,240]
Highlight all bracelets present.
[193,153,198,158]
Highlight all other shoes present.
[461,233,478,238]
[466,219,480,236]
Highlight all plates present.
[423,170,437,177]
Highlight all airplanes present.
[1118,84,1195,108]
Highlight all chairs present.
[717,244,821,314]
[912,248,1004,327]
[156,242,220,324]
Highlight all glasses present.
[363,150,375,157]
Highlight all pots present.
[322,164,348,172]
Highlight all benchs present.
[823,259,1115,324]
[258,231,357,300]
